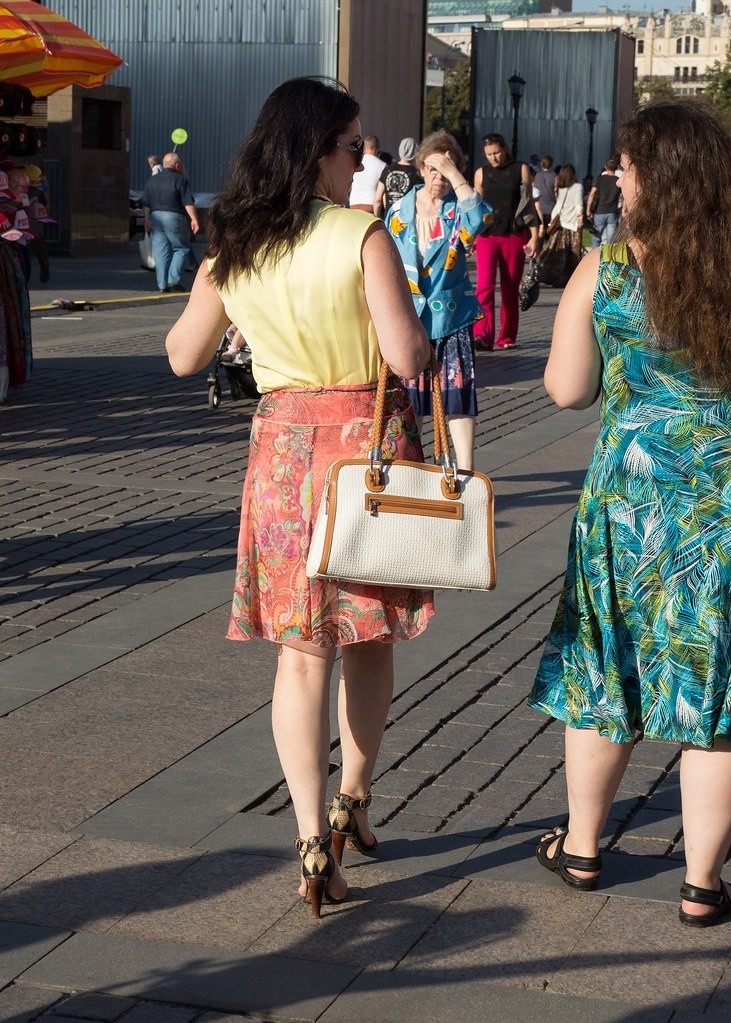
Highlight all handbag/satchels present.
[546,214,560,234]
[518,257,540,312]
[304,363,498,593]
[138,231,156,268]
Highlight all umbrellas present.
[0,0,129,100]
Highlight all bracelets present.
[454,182,469,190]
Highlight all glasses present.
[334,135,365,167]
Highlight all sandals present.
[536,827,602,888]
[678,877,731,927]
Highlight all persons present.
[222,323,247,361]
[162,75,432,919]
[139,151,200,294]
[349,132,628,353]
[524,98,731,928]
[384,131,493,473]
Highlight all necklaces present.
[313,195,330,202]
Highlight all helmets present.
[0,81,42,193]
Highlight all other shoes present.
[140,264,156,272]
[220,345,240,362]
[160,282,186,293]
[246,353,253,363]
[185,263,199,272]
[472,336,493,351]
[502,341,517,349]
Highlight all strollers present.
[207,327,261,410]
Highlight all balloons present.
[171,128,188,145]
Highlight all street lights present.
[584,107,598,194]
[509,70,526,160]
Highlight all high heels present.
[325,790,378,866]
[294,834,348,918]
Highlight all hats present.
[398,137,420,161]
[0,195,58,244]
